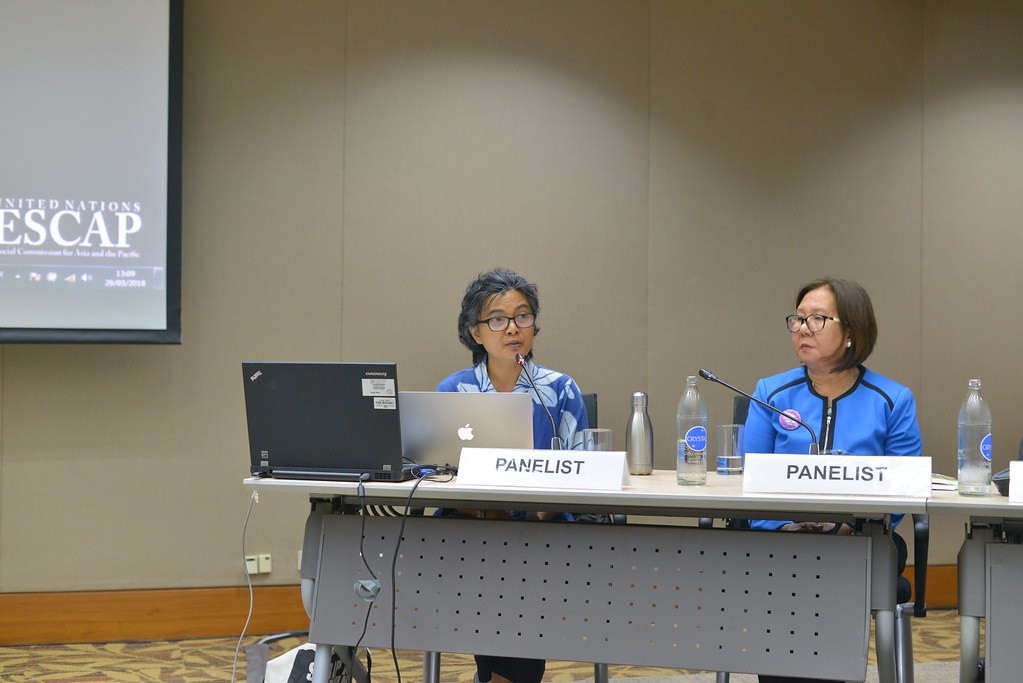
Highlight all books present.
[931,473,958,491]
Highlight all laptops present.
[398,391,533,468]
[241,362,438,483]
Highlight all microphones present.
[516,353,561,451]
[698,368,819,455]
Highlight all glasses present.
[786,313,841,333]
[476,313,537,332]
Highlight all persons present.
[736,277,922,683]
[437,272,593,683]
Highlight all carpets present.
[577,661,960,683]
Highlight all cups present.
[715,423,745,475]
[582,428,613,451]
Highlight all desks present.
[241,471,1022,683]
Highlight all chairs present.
[718,396,930,683]
[425,392,608,683]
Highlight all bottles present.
[625,391,654,476]
[957,378,993,497]
[675,375,708,486]
